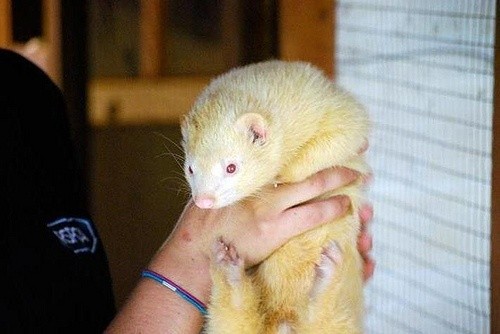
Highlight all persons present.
[0,44,376,334]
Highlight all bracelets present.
[139,269,209,318]
